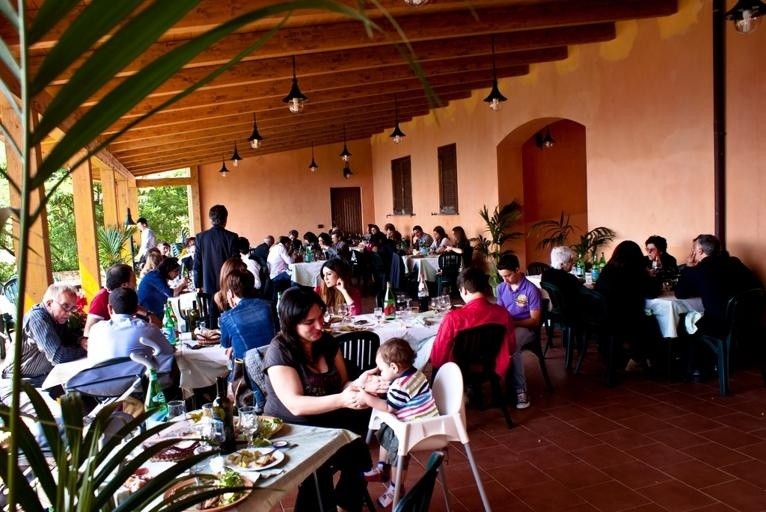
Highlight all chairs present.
[539,280,766,396]
[0,231,549,512]
[516,392,530,409]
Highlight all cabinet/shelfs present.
[598,251,609,271]
[415,239,427,255]
[295,242,322,263]
[417,274,429,313]
[181,262,189,278]
[143,367,167,423]
[189,298,207,341]
[591,253,601,286]
[401,237,407,249]
[161,300,178,348]
[384,281,396,320]
[575,251,586,280]
[212,375,236,454]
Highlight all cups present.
[57,302,74,311]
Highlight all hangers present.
[254,448,280,467]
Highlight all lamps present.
[230,141,242,168]
[725,2,765,33]
[340,126,352,160]
[248,112,263,151]
[219,153,229,177]
[309,141,318,172]
[389,100,406,143]
[484,34,507,111]
[282,55,308,114]
[344,162,353,179]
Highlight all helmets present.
[163,474,253,512]
[235,414,285,438]
[224,447,286,472]
[335,318,373,334]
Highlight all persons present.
[642,235,678,281]
[313,258,362,317]
[138,237,196,289]
[83,288,175,398]
[134,218,156,281]
[543,246,593,292]
[235,222,473,297]
[0,281,86,463]
[429,266,517,401]
[591,241,662,376]
[261,286,394,512]
[674,233,763,385]
[192,204,247,329]
[358,337,443,508]
[136,257,188,320]
[84,263,161,340]
[218,268,276,398]
[208,257,249,330]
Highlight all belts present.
[397,294,413,312]
[238,405,258,446]
[373,306,383,326]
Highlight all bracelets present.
[146,311,154,318]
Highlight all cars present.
[324,302,354,318]
[166,399,187,422]
[431,295,451,310]
[202,403,214,419]
